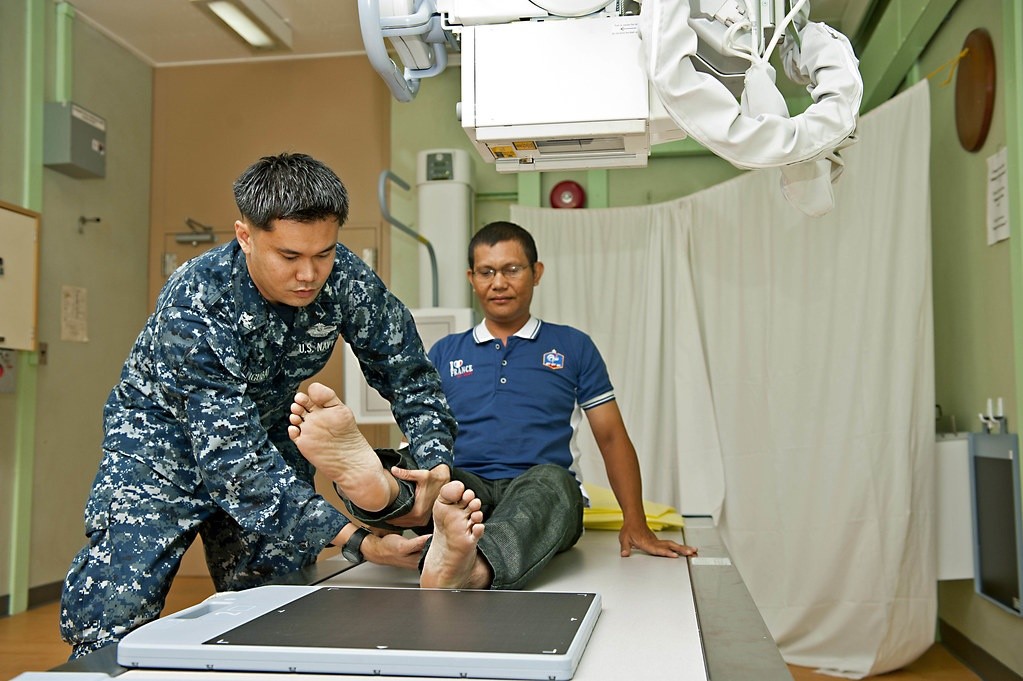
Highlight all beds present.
[8,516,793,680]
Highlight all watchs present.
[341,525,373,563]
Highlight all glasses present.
[469,261,535,280]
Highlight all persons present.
[287,222,697,591]
[59,152,459,661]
